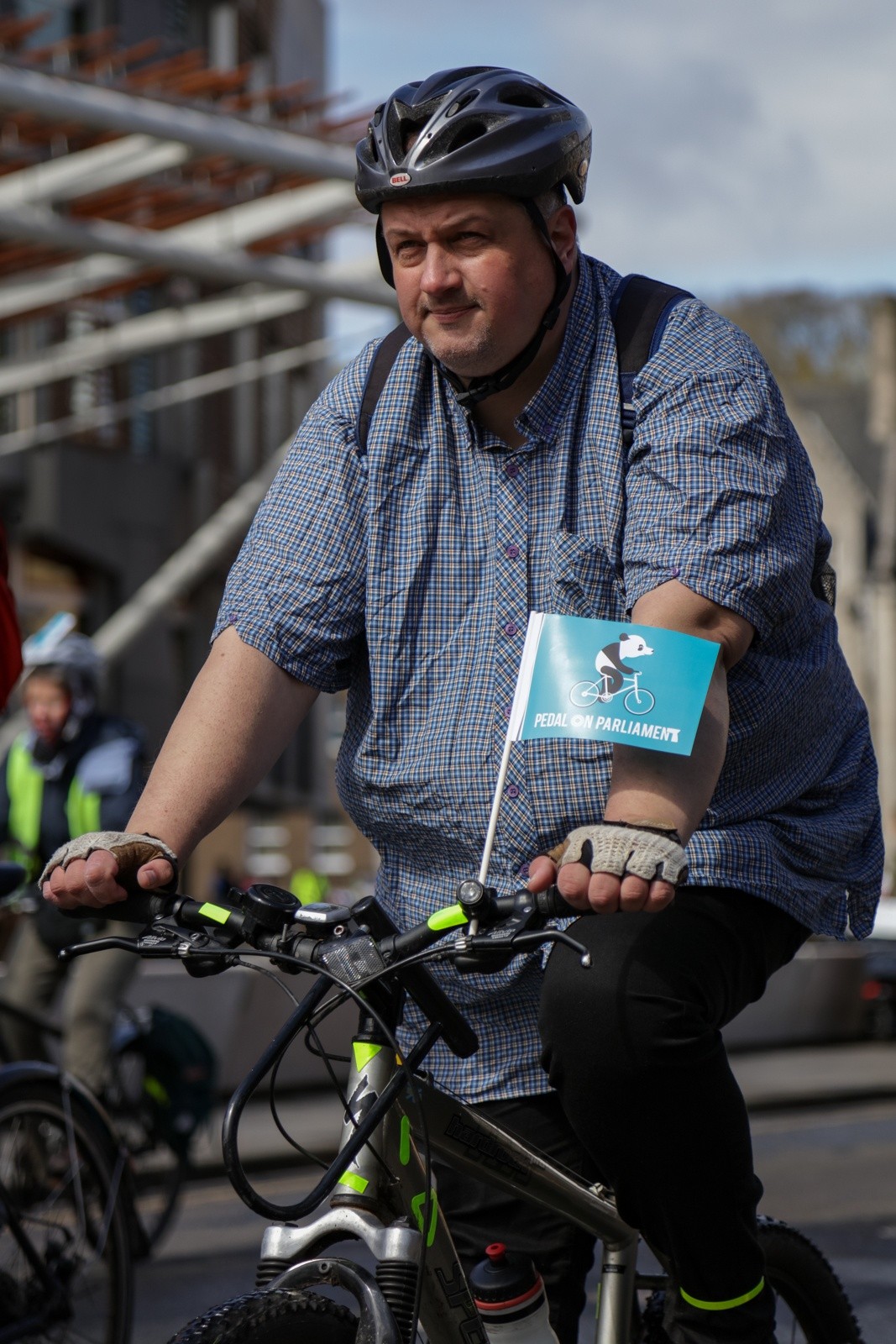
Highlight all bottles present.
[469,1243,559,1344]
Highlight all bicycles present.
[0,849,221,1344]
[54,869,871,1344]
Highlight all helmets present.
[352,66,593,214]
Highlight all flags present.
[507,613,720,756]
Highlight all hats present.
[23,609,98,667]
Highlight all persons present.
[0,640,144,1168]
[36,65,886,1344]
